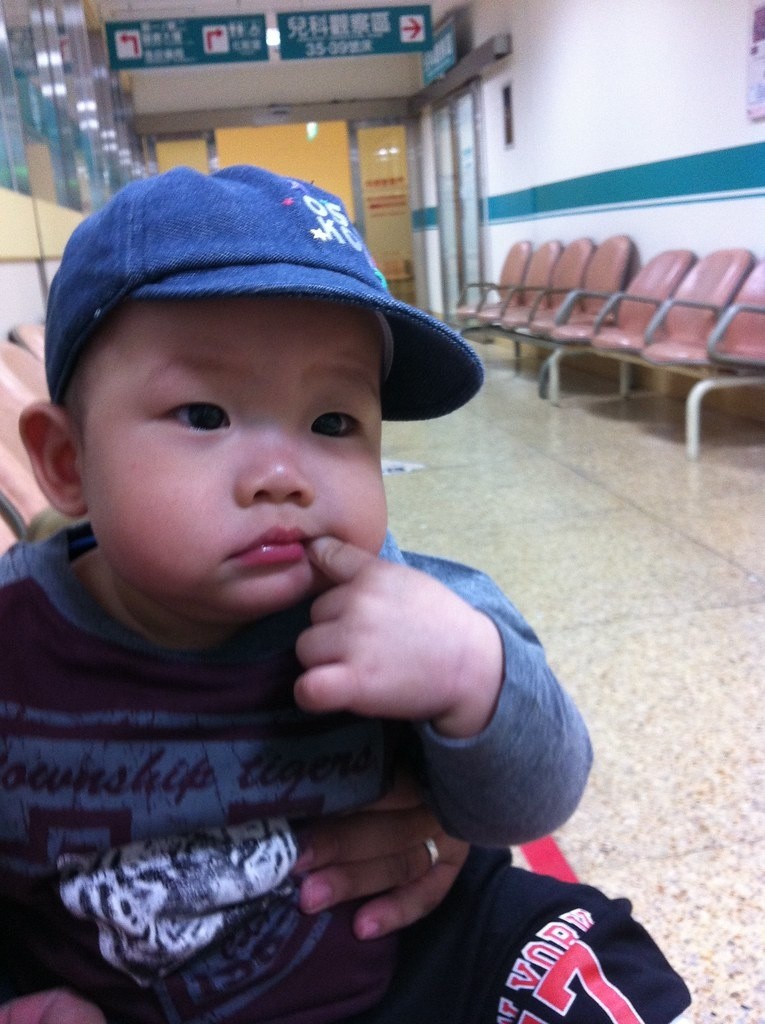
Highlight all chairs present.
[0,320,53,561]
[453,235,765,459]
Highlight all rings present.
[427,838,442,863]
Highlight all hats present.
[45,166,485,422]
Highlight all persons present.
[0,158,694,1024]
[1,772,472,1024]
